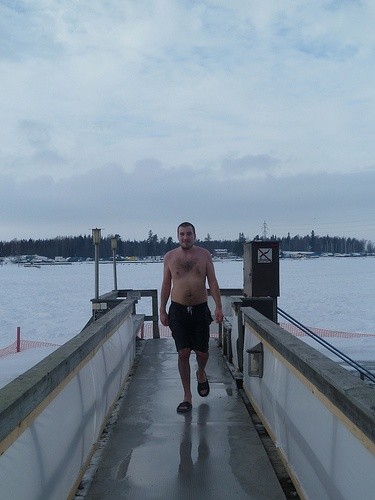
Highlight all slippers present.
[196,370,209,397]
[177,402,192,412]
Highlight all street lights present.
[111,238,117,290]
[92,228,102,299]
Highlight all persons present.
[160,222,224,412]
